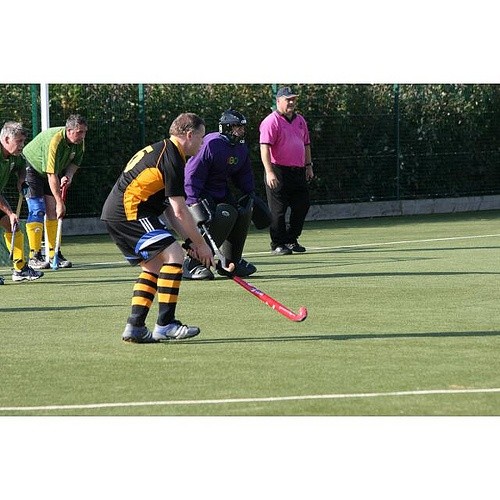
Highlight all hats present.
[276,86,299,99]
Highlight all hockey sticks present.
[202,224,236,272]
[52,176,69,271]
[182,243,309,322]
[9,192,24,261]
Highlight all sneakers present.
[218,259,257,276]
[272,246,292,255]
[182,259,215,279]
[28,252,50,269]
[152,320,200,340]
[284,242,306,253]
[0,277,4,285]
[12,264,43,281]
[122,322,160,343]
[49,250,72,268]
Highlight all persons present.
[23,113,89,270]
[0,120,45,284]
[258,86,316,257]
[98,113,218,344]
[181,110,273,279]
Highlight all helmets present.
[219,109,247,143]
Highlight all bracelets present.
[184,238,193,245]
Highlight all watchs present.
[305,162,313,167]
